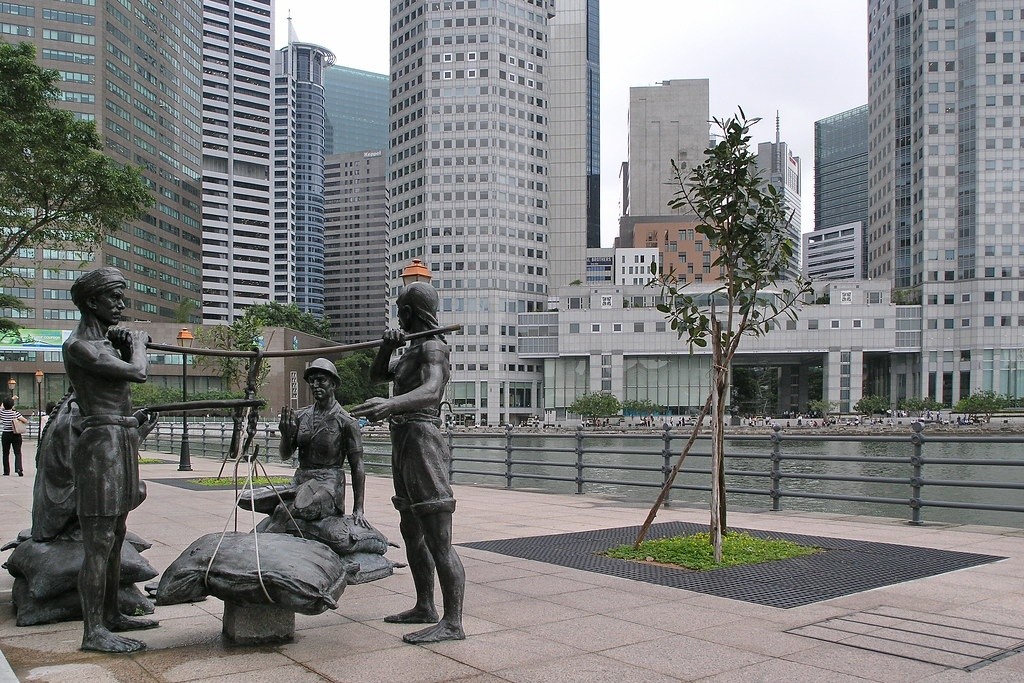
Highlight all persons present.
[581,412,712,427]
[40,401,56,440]
[62,266,159,653]
[0,394,27,477]
[30,380,159,543]
[911,406,989,428]
[347,281,465,644]
[740,408,910,428]
[237,358,372,530]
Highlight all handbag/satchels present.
[12,419,27,434]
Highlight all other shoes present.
[16,469,23,475]
[4,472,9,476]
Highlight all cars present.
[32,409,46,417]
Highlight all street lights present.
[35,369,43,447]
[9,378,16,399]
[177,328,195,470]
